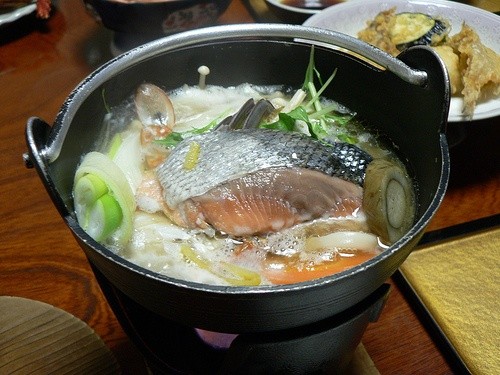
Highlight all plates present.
[0,0,55,40]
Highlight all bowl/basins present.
[264,0,344,27]
[84,0,234,53]
[293,1,500,132]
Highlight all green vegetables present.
[155,43,360,148]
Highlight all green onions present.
[72,172,122,247]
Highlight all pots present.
[22,23,451,336]
